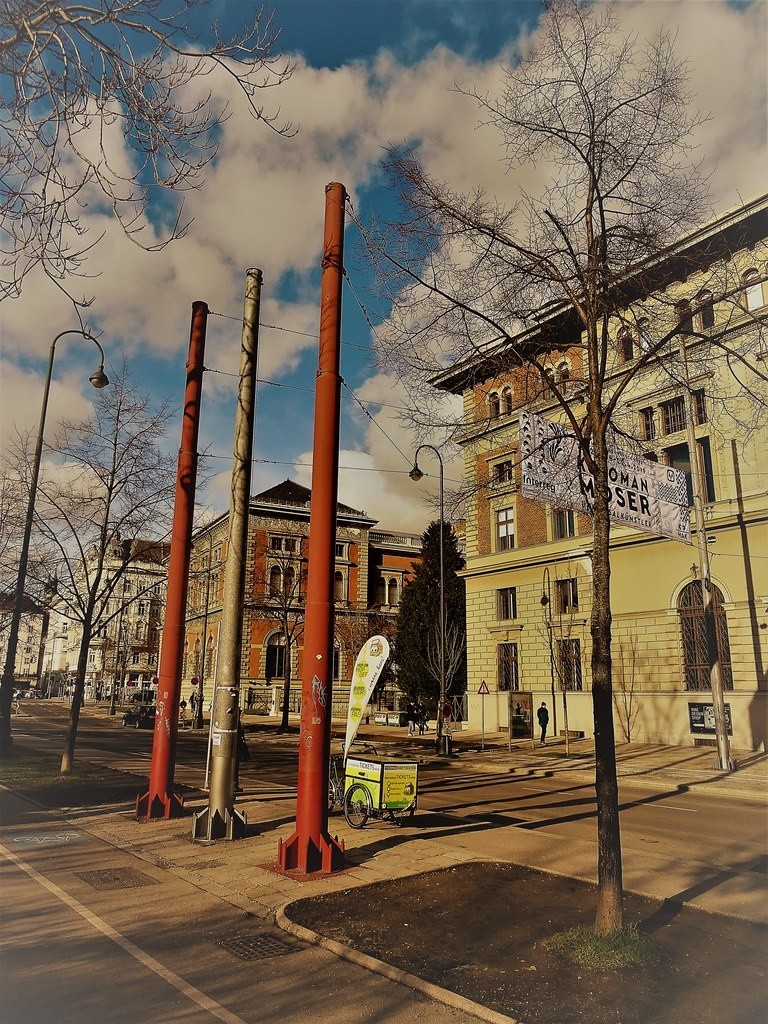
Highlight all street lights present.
[190,527,212,729]
[410,445,459,758]
[540,567,556,736]
[0,331,109,756]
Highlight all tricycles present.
[328,742,420,829]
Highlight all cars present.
[122,705,156,729]
[14,689,49,700]
[129,693,147,703]
